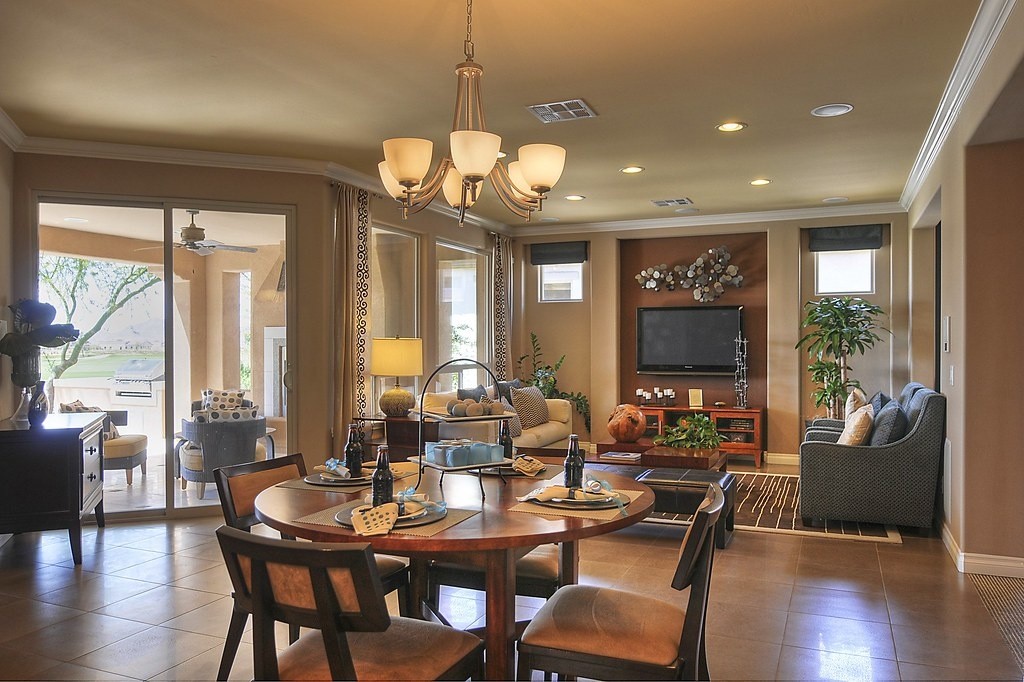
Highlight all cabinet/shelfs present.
[636,403,763,469]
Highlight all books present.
[599,451,642,462]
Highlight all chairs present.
[427,448,586,612]
[216,525,486,682]
[104,434,148,484]
[173,398,267,500]
[215,452,409,646]
[513,482,726,682]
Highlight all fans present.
[134,210,259,256]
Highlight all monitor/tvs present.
[635,305,744,375]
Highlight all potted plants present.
[0,298,80,388]
[794,296,893,427]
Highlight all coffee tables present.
[583,437,729,473]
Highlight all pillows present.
[871,398,908,447]
[868,390,892,416]
[184,409,208,450]
[58,399,82,413]
[509,385,550,430]
[205,387,246,408]
[480,395,522,438]
[493,377,522,406]
[207,405,259,424]
[834,403,874,447]
[201,389,207,409]
[78,406,120,441]
[457,384,488,403]
[845,388,867,427]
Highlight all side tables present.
[352,415,442,463]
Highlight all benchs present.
[584,464,734,551]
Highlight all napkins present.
[350,503,398,536]
[512,455,547,477]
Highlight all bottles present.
[501,421,513,459]
[345,424,362,477]
[563,434,583,488]
[372,445,393,507]
[28,381,48,425]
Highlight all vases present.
[28,380,48,427]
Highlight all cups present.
[425,439,504,466]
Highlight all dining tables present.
[255,462,655,682]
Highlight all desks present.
[0,412,109,566]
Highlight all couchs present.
[416,386,572,448]
[800,382,946,538]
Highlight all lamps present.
[377,0,566,227]
[370,335,425,418]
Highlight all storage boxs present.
[641,447,719,469]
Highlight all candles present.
[635,386,676,400]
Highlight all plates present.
[320,469,369,480]
[552,493,614,503]
[351,502,426,519]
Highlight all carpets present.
[641,471,904,544]
